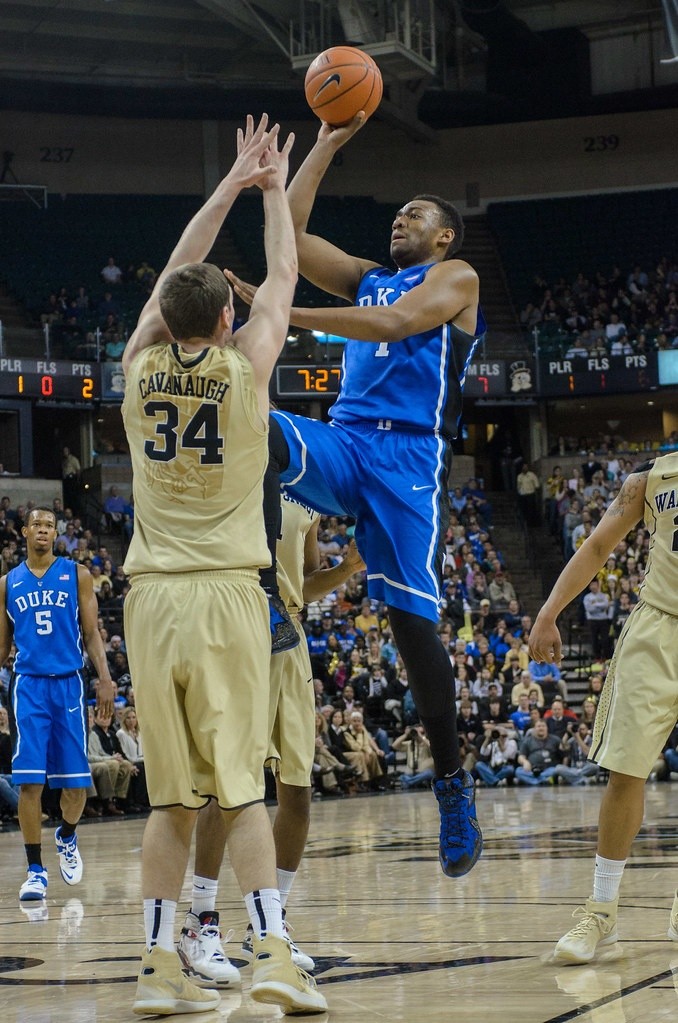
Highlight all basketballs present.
[304,46,383,127]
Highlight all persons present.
[1,506,115,903]
[114,109,330,1018]
[1,431,678,821]
[38,250,159,362]
[234,109,493,877]
[521,251,678,361]
[172,391,368,993]
[529,451,677,964]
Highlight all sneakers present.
[19,864,48,899]
[55,825,82,887]
[248,931,331,1015]
[176,909,241,990]
[133,945,222,1014]
[431,770,482,877]
[554,896,622,963]
[242,907,315,972]
[667,890,678,941]
